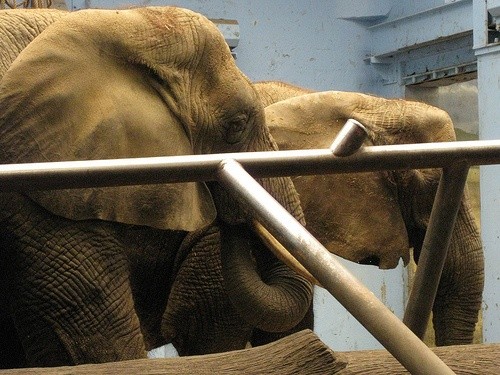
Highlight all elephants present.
[0,5,313,367]
[251,77,483,348]
